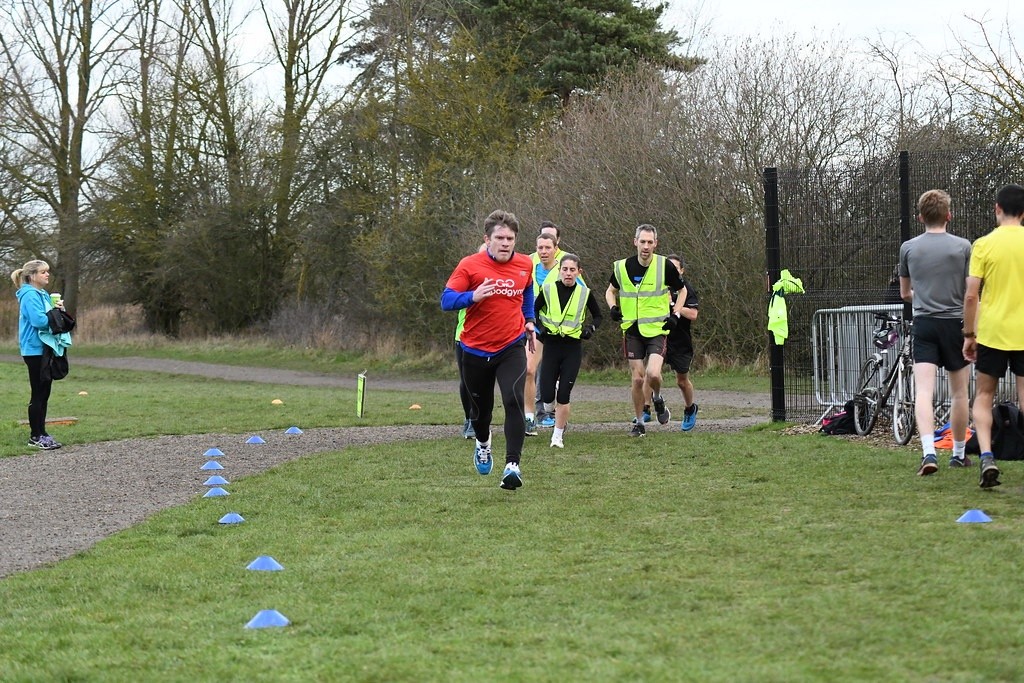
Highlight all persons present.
[455,308,476,439]
[442,210,537,490]
[899,190,973,476]
[962,185,1024,487]
[633,255,699,430]
[11,260,65,449]
[605,224,688,437]
[525,222,603,447]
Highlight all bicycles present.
[853,312,916,445]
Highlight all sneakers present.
[916,453,938,477]
[43,435,61,449]
[536,410,555,428]
[978,456,1002,488]
[550,433,564,449]
[500,462,522,490]
[544,381,560,412]
[524,418,539,436]
[633,411,652,423]
[462,420,476,440]
[473,430,493,476]
[651,391,671,425]
[627,424,646,438]
[681,404,698,430]
[27,435,57,450]
[948,455,977,468]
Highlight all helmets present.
[872,326,899,349]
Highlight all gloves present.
[609,306,623,321]
[662,314,679,330]
[538,326,551,339]
[580,323,596,340]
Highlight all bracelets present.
[963,331,974,337]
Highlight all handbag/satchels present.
[49,350,68,380]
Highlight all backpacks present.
[965,402,1024,460]
[824,400,868,435]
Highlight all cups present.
[50,293,61,309]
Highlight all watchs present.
[670,302,675,308]
[526,318,536,325]
